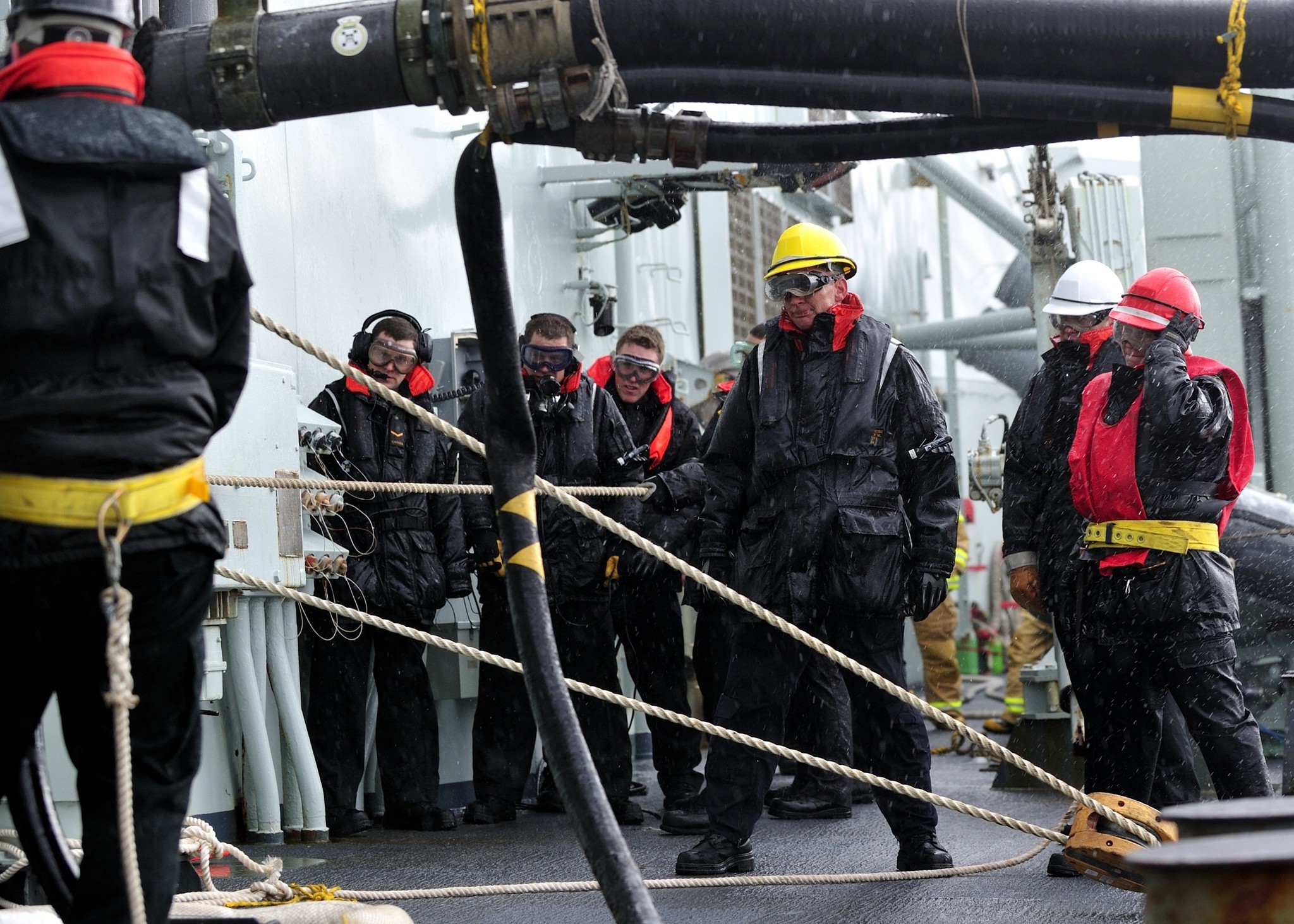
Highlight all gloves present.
[470,521,1050,626]
[1157,309,1201,356]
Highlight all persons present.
[1049,267,1274,874]
[0,2,255,924]
[600,324,706,825]
[650,323,1060,836]
[1000,258,1209,876]
[297,316,474,837]
[455,312,647,826]
[675,221,958,877]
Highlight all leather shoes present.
[327,714,1123,879]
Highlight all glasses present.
[1047,308,1112,334]
[519,335,575,372]
[368,338,419,375]
[610,351,663,385]
[1111,320,1163,351]
[763,269,843,304]
[729,340,757,368]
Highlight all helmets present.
[5,0,137,49]
[763,222,858,281]
[1108,266,1206,342]
[1042,259,1127,317]
